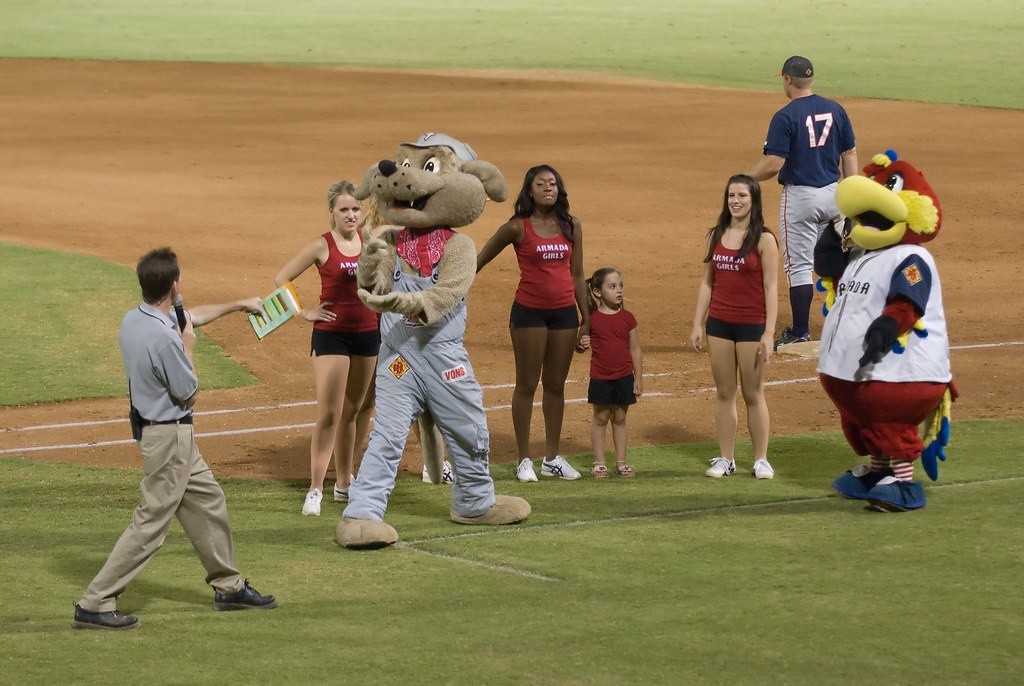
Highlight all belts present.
[140,416,194,424]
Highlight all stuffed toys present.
[814,149,959,512]
[335,132,533,546]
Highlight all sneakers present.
[705,457,736,477]
[334,483,352,500]
[540,455,582,480]
[302,488,324,515]
[515,457,538,482]
[753,458,775,479]
[773,328,811,351]
[422,460,456,484]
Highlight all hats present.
[776,56,814,78]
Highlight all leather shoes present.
[72,604,141,628]
[214,579,276,610]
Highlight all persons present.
[274,180,454,516]
[72,247,277,630]
[748,55,858,350]
[690,174,780,479]
[575,267,643,477]
[475,164,589,483]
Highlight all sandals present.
[590,462,609,477]
[617,464,634,478]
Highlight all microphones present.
[174,293,187,333]
